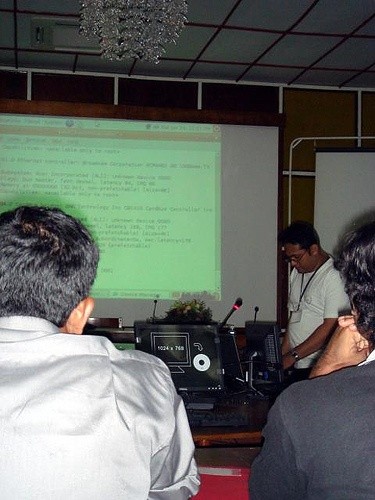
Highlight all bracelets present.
[290,349,301,361]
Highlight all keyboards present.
[187,413,268,428]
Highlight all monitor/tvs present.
[133,322,226,410]
[242,321,284,388]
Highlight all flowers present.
[166,298,213,321]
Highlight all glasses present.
[281,249,309,263]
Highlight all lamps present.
[78,0,188,64]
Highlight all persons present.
[0,206,201,500]
[278,221,353,382]
[249,218,375,500]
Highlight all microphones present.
[151,300,158,325]
[221,300,243,325]
[254,307,259,322]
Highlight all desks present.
[187,394,281,448]
